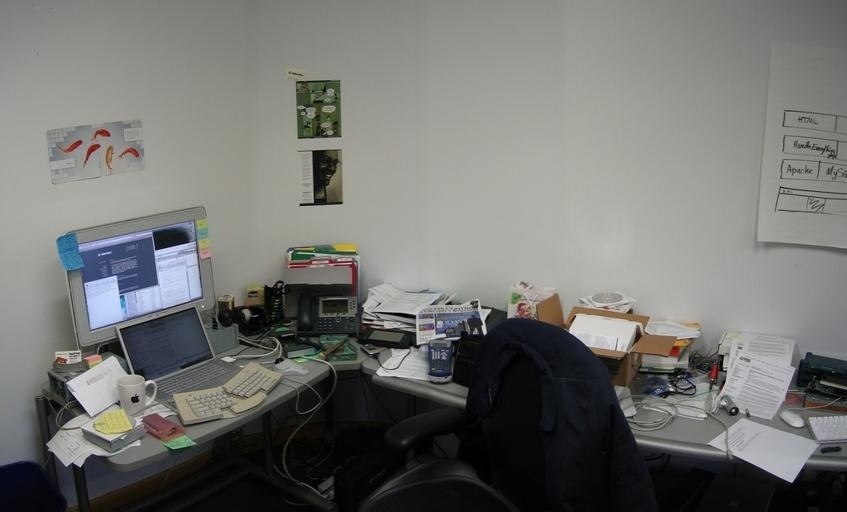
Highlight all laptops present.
[113,301,243,414]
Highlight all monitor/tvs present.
[65,205,216,351]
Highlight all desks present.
[35,295,847,511]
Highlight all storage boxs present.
[536,293,678,386]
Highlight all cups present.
[117,374,157,416]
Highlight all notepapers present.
[84,354,102,369]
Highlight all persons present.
[312,150,342,203]
[467,314,484,336]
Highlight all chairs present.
[357,316,655,511]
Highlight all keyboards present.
[807,415,847,444]
[171,361,285,426]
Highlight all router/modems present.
[81,408,145,454]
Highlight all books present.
[567,313,644,354]
[637,319,703,374]
[373,303,416,326]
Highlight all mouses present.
[272,357,310,376]
[780,410,805,428]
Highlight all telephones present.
[297,294,358,336]
